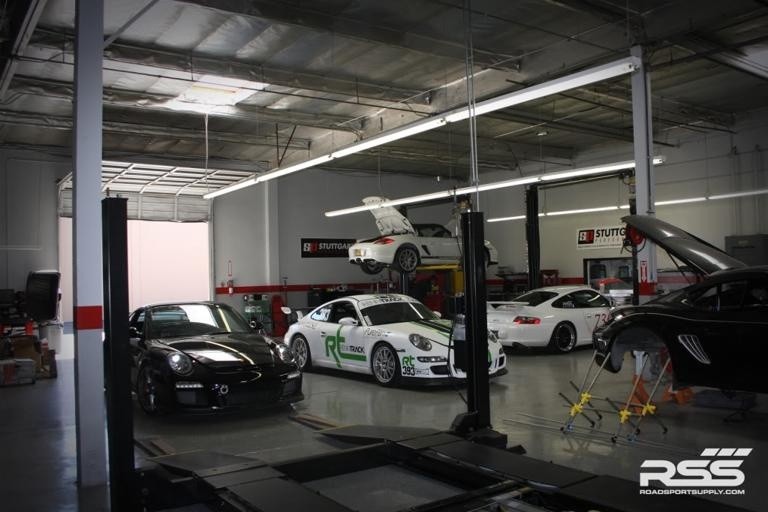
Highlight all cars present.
[592,207,767,403]
[104,300,308,420]
[346,197,500,277]
[276,289,510,389]
[486,283,624,356]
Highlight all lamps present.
[204,0,768,223]
